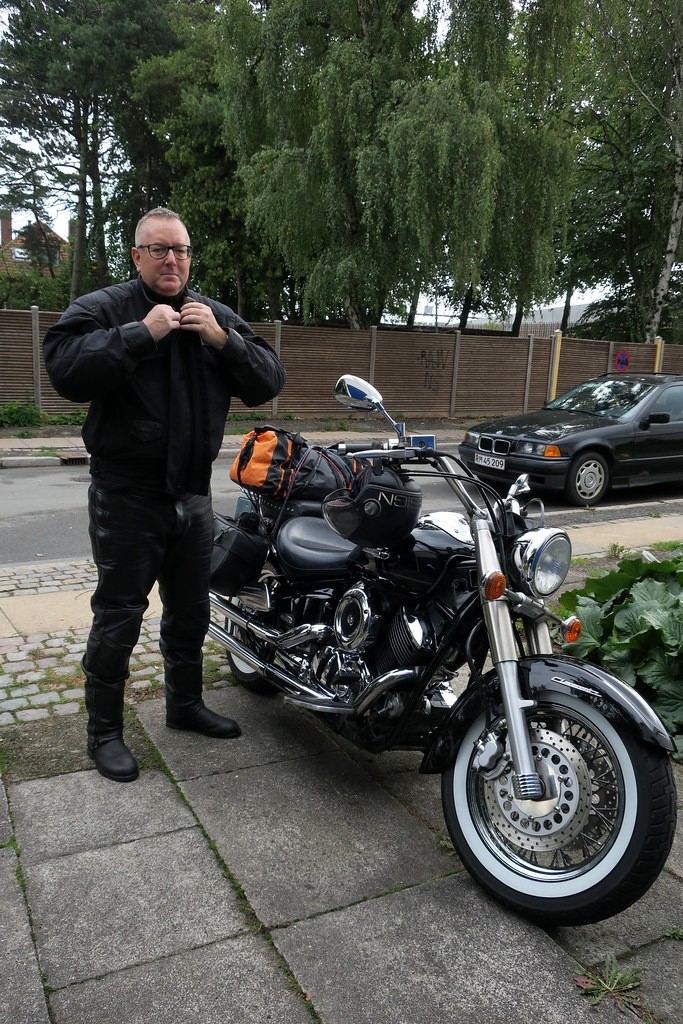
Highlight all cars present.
[458,370,683,505]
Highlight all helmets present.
[321,467,421,548]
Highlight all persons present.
[42,205,288,782]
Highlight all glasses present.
[137,243,192,261]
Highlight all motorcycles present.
[205,375,680,926]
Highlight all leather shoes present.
[87,739,138,782]
[165,703,242,738]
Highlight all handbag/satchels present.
[229,424,372,501]
